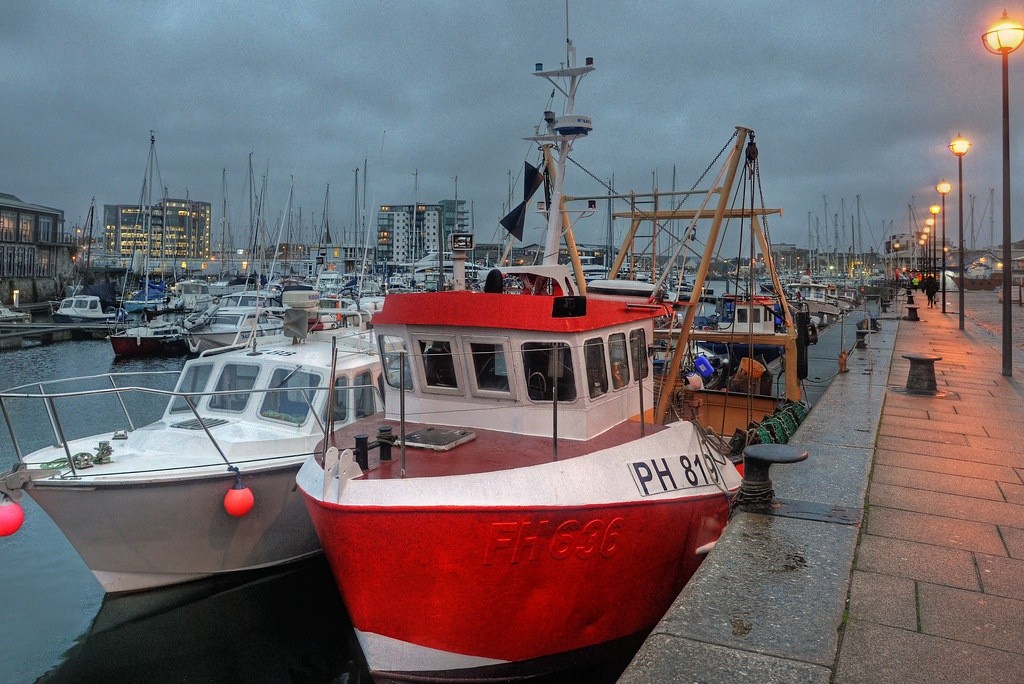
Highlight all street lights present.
[892,179,950,313]
[948,132,972,332]
[980,8,1023,375]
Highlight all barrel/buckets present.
[694,356,714,377]
[755,354,770,373]
[735,358,766,381]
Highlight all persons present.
[910,271,939,308]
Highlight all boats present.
[0,228,476,598]
[53,123,880,360]
[293,39,811,681]
